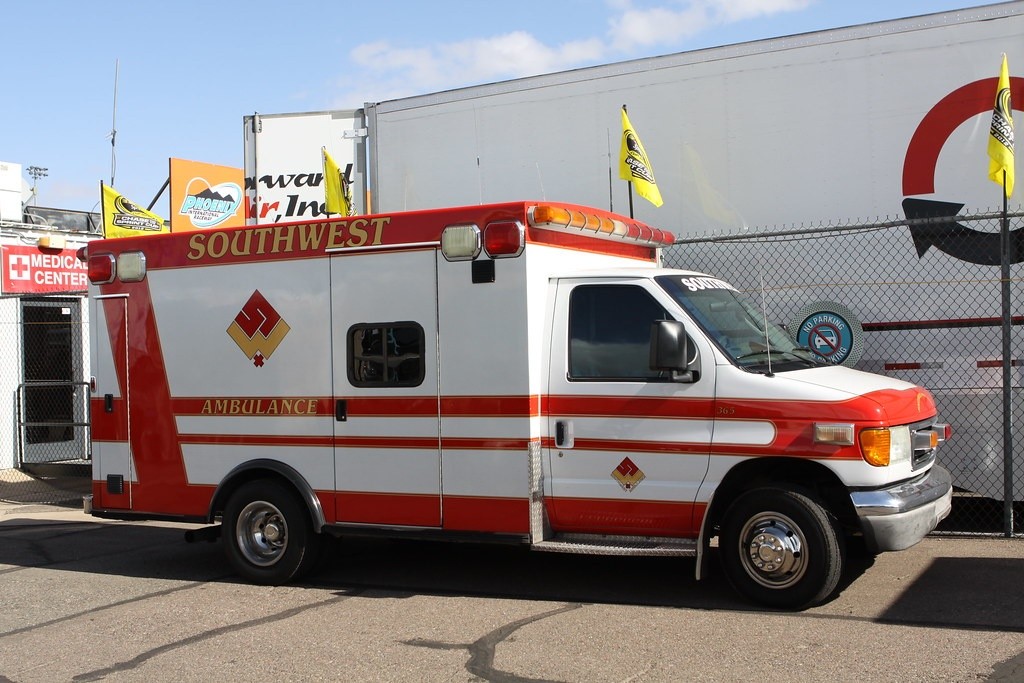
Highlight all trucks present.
[242,0,1023,502]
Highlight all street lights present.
[25,165,48,206]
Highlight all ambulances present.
[82,201,954,616]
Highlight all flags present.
[101,180,168,239]
[322,146,358,219]
[986,54,1016,200]
[618,107,665,208]
